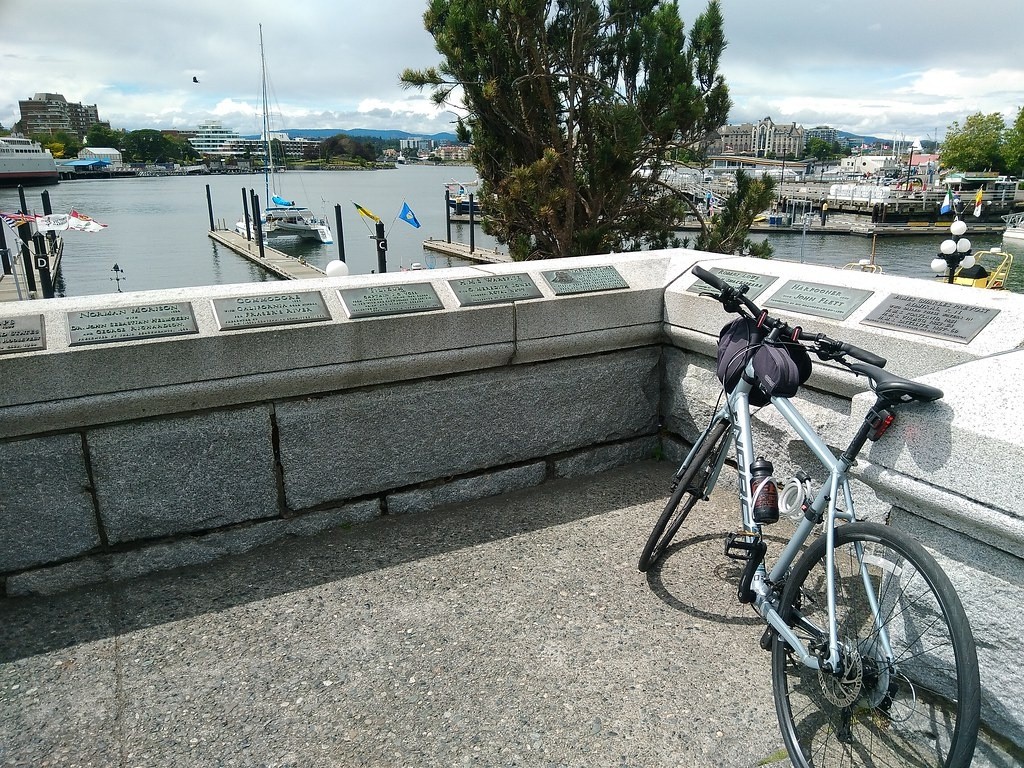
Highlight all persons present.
[953,189,965,221]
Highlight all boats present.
[442,174,482,214]
[0,133,59,188]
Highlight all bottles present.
[749,456,780,524]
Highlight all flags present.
[940,187,953,214]
[863,144,869,149]
[35,213,70,232]
[0,209,35,229]
[399,202,422,229]
[354,202,380,223]
[883,146,889,150]
[973,188,983,217]
[65,210,109,232]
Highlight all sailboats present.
[234,22,335,251]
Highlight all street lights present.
[930,221,975,284]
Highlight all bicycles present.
[638,265,982,768]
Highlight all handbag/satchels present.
[715,308,812,408]
[752,344,799,398]
